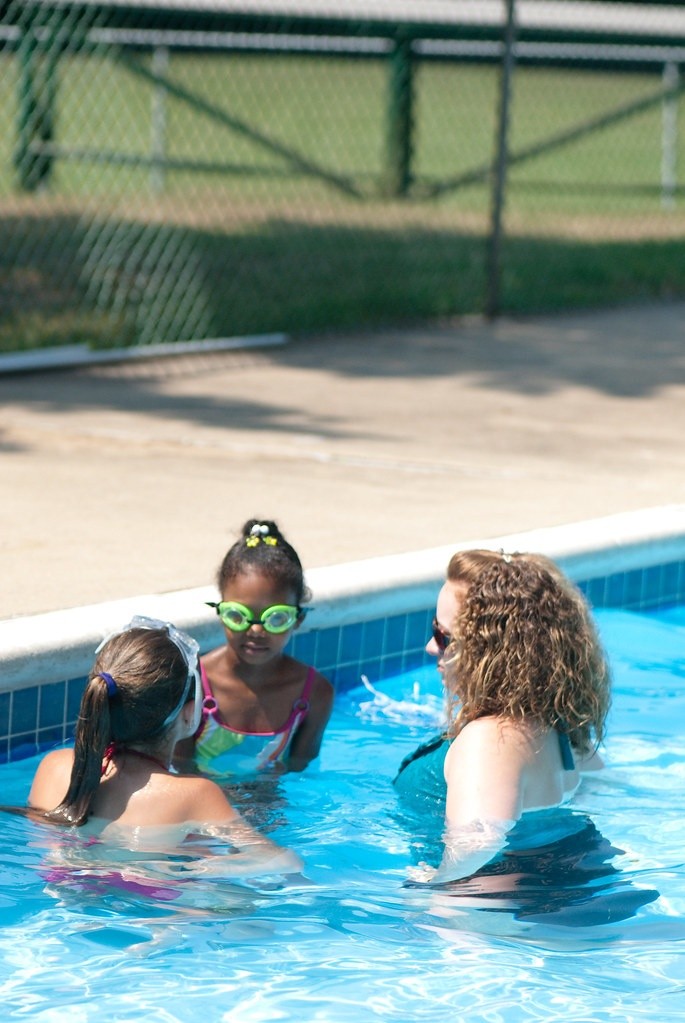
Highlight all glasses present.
[427,613,459,653]
[93,615,199,723]
[214,596,303,631]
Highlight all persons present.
[21,614,302,875]
[392,545,609,884]
[169,518,335,783]
